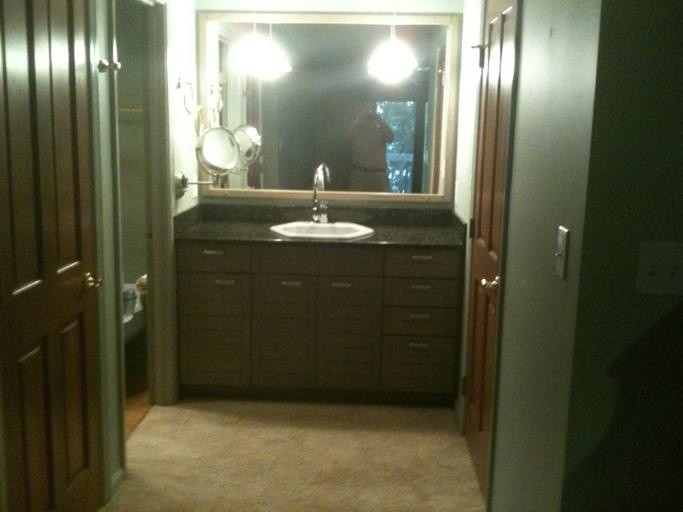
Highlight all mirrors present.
[233,123,262,176]
[197,9,462,202]
[176,127,240,202]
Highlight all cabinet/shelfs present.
[250,243,314,394]
[180,242,250,394]
[382,245,463,399]
[314,245,382,396]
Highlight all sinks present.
[269,219,376,242]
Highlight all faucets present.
[313,163,331,188]
[312,200,333,222]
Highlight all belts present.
[354,166,386,172]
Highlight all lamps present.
[366,14,420,88]
[225,12,296,87]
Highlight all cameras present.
[366,110,373,117]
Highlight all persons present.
[340,97,394,192]
